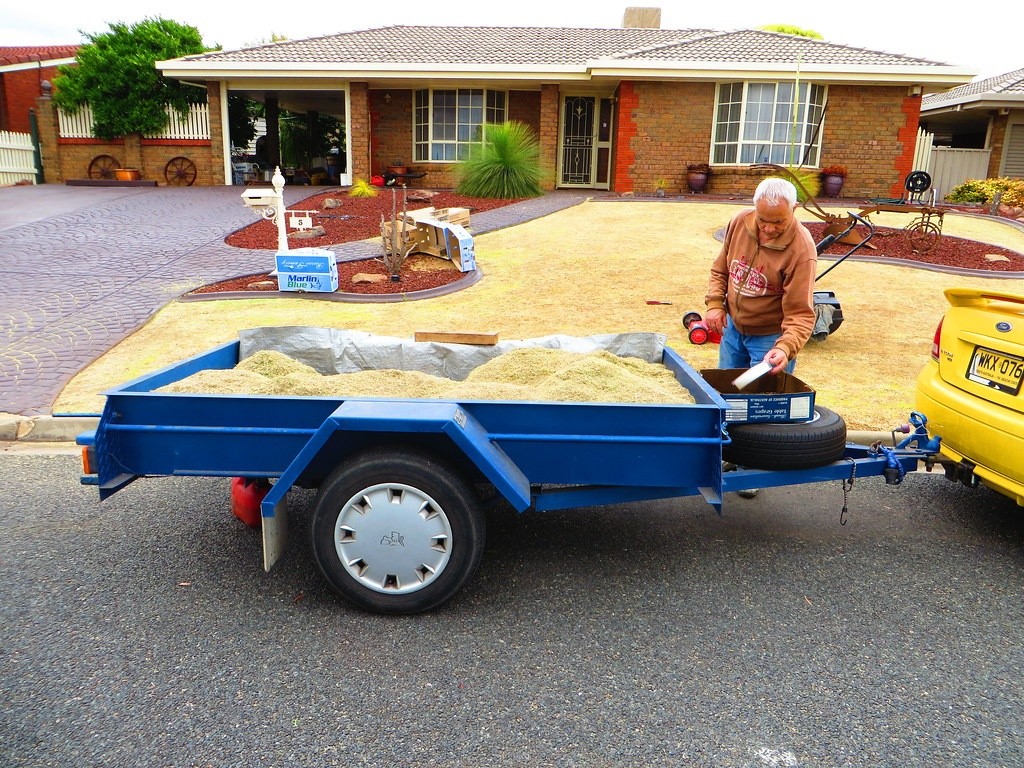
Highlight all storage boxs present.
[234,163,273,181]
[698,368,817,424]
[275,251,339,293]
[380,206,476,272]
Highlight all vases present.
[657,188,664,197]
[688,171,707,192]
[823,174,843,196]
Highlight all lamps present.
[383,93,392,104]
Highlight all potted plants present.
[114,167,139,180]
[386,162,407,185]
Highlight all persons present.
[705,178,817,498]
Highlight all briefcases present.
[371,175,385,187]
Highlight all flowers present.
[687,164,712,176]
[821,164,848,178]
[656,179,666,188]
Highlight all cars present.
[914,286,1024,507]
